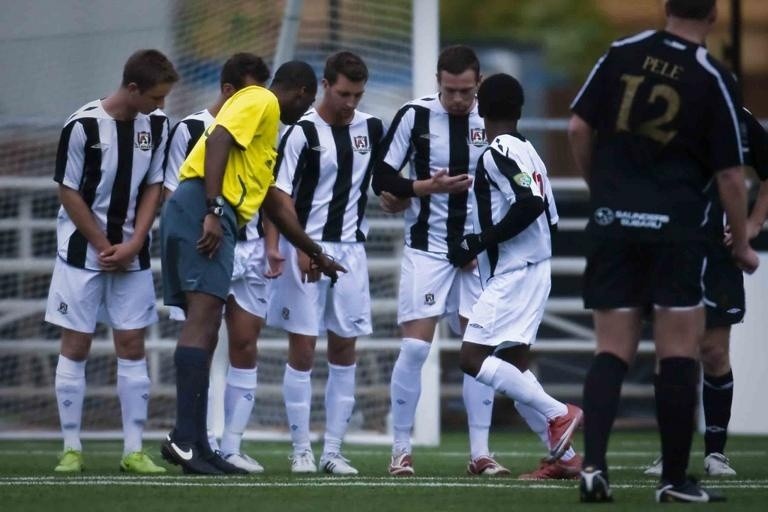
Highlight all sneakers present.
[579,466,613,502]
[518,451,584,481]
[53,449,84,473]
[219,451,264,474]
[289,443,318,474]
[120,450,166,474]
[546,402,585,459]
[388,455,416,477]
[655,473,726,502]
[160,438,222,476]
[654,477,735,491]
[643,455,663,476]
[702,452,737,477]
[319,451,359,476]
[204,449,237,474]
[466,452,511,476]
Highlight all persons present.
[159,61,348,475]
[262,51,413,475]
[444,72,584,480]
[568,0,758,504]
[367,44,513,476]
[162,51,286,473]
[43,50,180,474]
[644,107,767,477]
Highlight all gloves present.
[445,233,483,270]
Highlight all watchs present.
[206,195,225,207]
[206,207,225,217]
